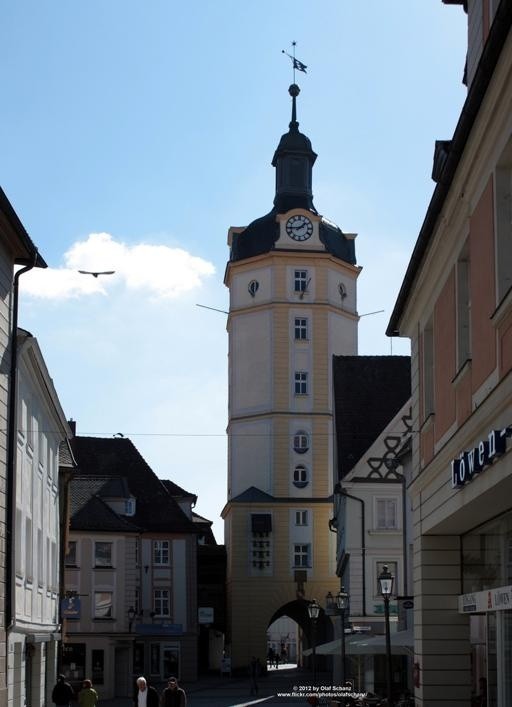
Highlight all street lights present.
[375,563,400,706]
[335,585,351,706]
[306,597,322,706]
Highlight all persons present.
[248,653,265,695]
[218,657,228,681]
[131,675,161,706]
[78,678,99,706]
[51,673,75,706]
[157,675,188,706]
[281,645,288,663]
[267,647,276,668]
[275,653,280,668]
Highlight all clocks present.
[286,215,314,241]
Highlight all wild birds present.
[78,270,115,278]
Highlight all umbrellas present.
[302,629,414,691]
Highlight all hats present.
[80,680,91,686]
[168,677,177,684]
[57,674,65,680]
